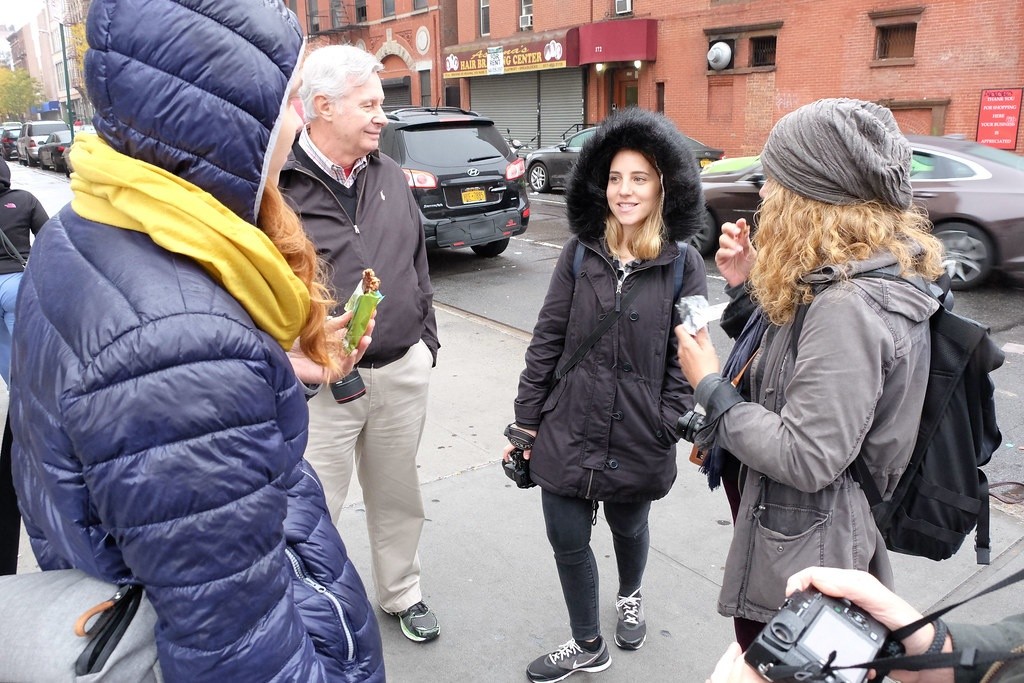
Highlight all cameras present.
[330,365,366,405]
[502,447,538,489]
[747,583,907,683]
[674,410,707,444]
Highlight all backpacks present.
[790,262,1006,565]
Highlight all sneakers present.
[380,600,440,641]
[614,586,647,649]
[526,634,612,683]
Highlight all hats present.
[761,96,913,213]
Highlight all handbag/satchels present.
[0,568,165,683]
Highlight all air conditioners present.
[615,0,632,14]
[520,14,533,28]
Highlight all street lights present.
[59,22,77,142]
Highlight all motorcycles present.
[505,128,536,160]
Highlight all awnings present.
[31,101,59,114]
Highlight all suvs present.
[16,119,70,166]
[378,106,530,258]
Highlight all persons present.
[674,98,939,654]
[278,44,441,644]
[705,566,1024,683]
[9,0,386,683]
[503,103,709,683]
[0,155,48,392]
[74,119,81,126]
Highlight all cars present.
[0,121,24,161]
[525,123,724,193]
[38,130,72,171]
[681,137,1024,291]
